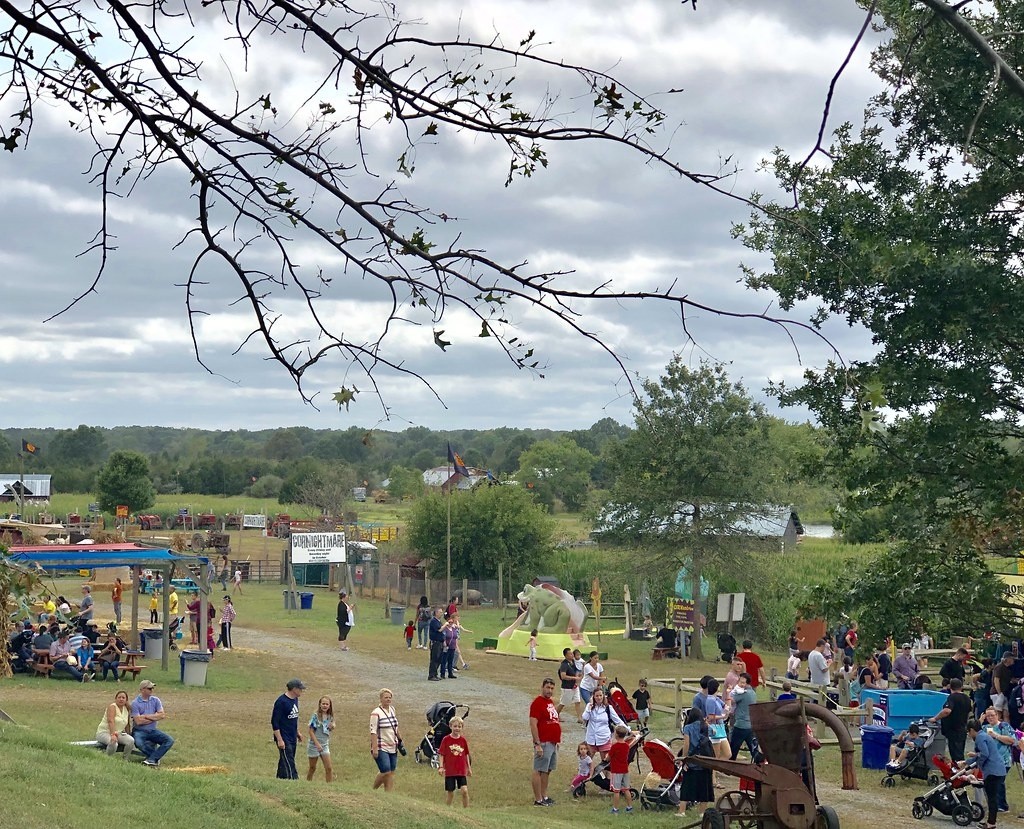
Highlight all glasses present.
[987,714,995,718]
[904,648,910,650]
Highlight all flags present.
[448,442,468,476]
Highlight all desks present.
[35,634,145,679]
[140,578,198,595]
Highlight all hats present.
[1003,651,1014,658]
[82,585,91,592]
[288,679,307,689]
[140,680,156,689]
[902,643,912,649]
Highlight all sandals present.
[713,783,726,789]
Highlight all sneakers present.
[624,807,634,813]
[609,808,620,814]
[534,800,550,807]
[543,797,556,804]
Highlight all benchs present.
[139,585,200,595]
[651,646,688,660]
[12,656,148,681]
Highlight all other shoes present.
[885,759,901,770]
[407,644,470,682]
[696,813,704,817]
[143,760,156,765]
[997,810,1011,813]
[976,822,996,828]
[674,812,685,817]
[155,759,161,765]
[81,673,88,683]
[89,673,95,680]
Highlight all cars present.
[354,492,366,502]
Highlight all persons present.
[271,594,1024,829]
[5,554,243,766]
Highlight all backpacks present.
[850,667,867,695]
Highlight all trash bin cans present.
[925,725,946,769]
[179,649,212,687]
[283,590,299,609]
[390,607,405,625]
[139,628,163,659]
[300,592,314,609]
[861,724,894,770]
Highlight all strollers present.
[168,616,185,653]
[716,630,738,662]
[912,753,986,826]
[573,727,651,800]
[414,701,471,771]
[881,719,941,787]
[640,736,701,811]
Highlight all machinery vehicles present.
[192,531,231,555]
[273,513,291,539]
[219,511,242,529]
[193,511,217,530]
[140,514,163,530]
[166,511,192,530]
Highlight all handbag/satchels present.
[398,742,407,757]
[67,653,77,665]
[97,652,115,664]
[209,604,216,618]
